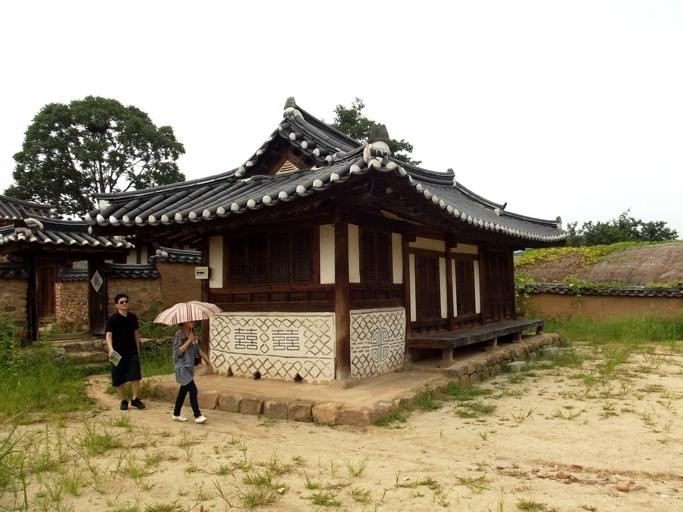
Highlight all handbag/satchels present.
[194,357,199,366]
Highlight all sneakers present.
[120,398,145,410]
[172,412,208,423]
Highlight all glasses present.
[119,300,129,304]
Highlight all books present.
[107,350,121,367]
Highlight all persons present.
[104,293,145,409]
[170,320,211,422]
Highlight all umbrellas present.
[151,301,222,344]
